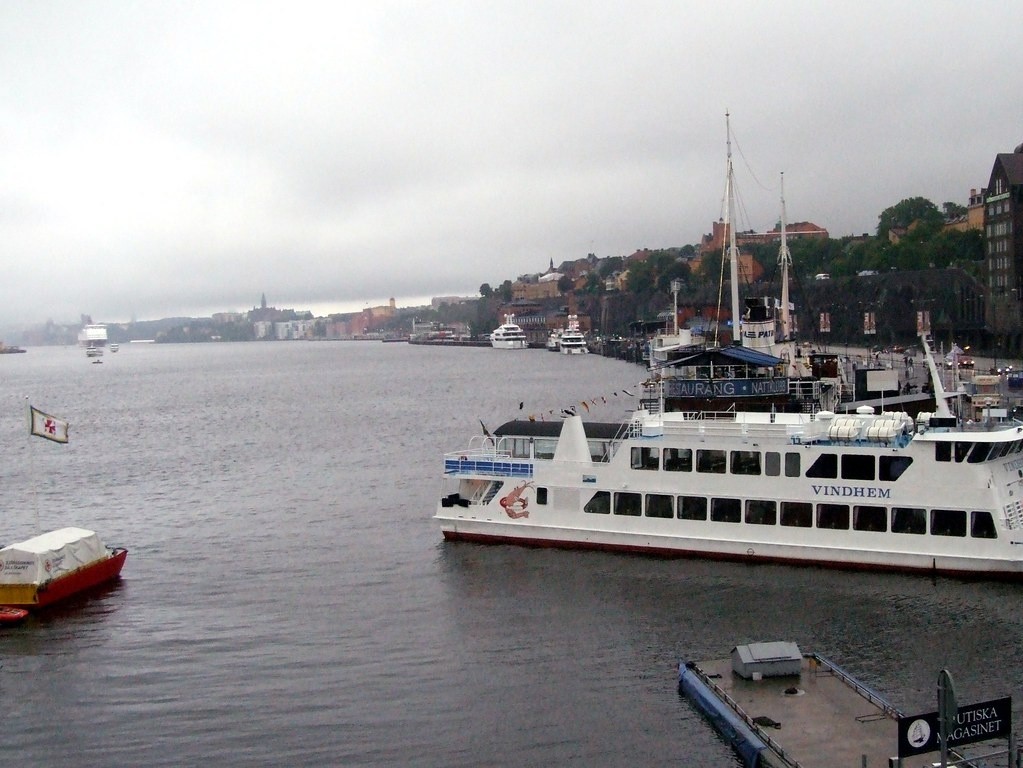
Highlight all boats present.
[0,527,128,626]
[0,341,121,366]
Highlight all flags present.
[28,404,68,443]
[820,312,831,332]
[916,310,932,336]
[529,389,634,423]
[789,315,799,333]
[864,312,877,335]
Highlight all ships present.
[77,313,108,347]
[382,107,1023,583]
